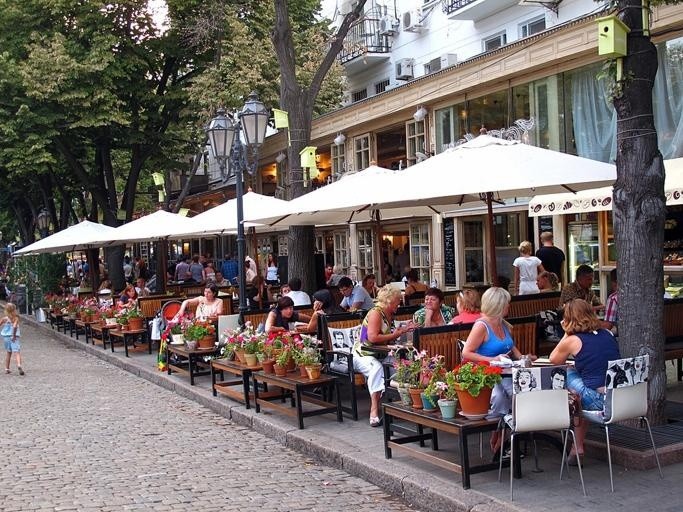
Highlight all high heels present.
[568,452,584,468]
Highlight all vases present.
[273,364,288,377]
[454,383,492,414]
[116,325,122,331]
[421,393,439,411]
[409,388,424,409]
[398,388,413,406]
[305,363,322,379]
[298,363,308,378]
[89,314,99,322]
[235,349,248,364]
[197,336,216,348]
[124,325,129,331]
[86,316,90,322]
[184,340,197,351]
[81,308,85,311]
[244,354,256,367]
[172,334,185,342]
[272,349,283,361]
[256,354,266,363]
[228,349,235,361]
[284,358,296,373]
[81,317,86,322]
[129,317,143,330]
[100,320,106,326]
[438,399,458,418]
[103,320,107,325]
[262,360,275,375]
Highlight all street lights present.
[36,208,51,239]
[205,90,270,330]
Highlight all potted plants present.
[44,293,83,316]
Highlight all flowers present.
[281,332,293,358]
[229,336,245,351]
[113,309,123,324]
[444,371,456,400]
[86,298,98,314]
[408,346,429,390]
[258,332,266,354]
[276,328,284,349]
[186,327,195,342]
[301,334,323,366]
[289,335,304,364]
[244,320,259,354]
[100,305,108,320]
[182,312,196,336]
[81,296,86,308]
[224,328,240,358]
[125,298,144,320]
[421,354,447,407]
[166,315,184,334]
[195,321,215,340]
[96,312,103,320]
[265,332,278,361]
[276,348,290,368]
[80,311,85,317]
[119,318,128,325]
[392,359,413,388]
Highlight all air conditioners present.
[403,9,419,31]
[379,15,392,35]
[395,58,412,79]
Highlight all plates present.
[458,410,490,420]
[171,342,186,346]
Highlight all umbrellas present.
[172,190,293,279]
[13,220,115,282]
[238,159,503,290]
[105,209,190,287]
[364,126,616,284]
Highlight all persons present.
[632,356,647,387]
[95,259,104,284]
[414,289,457,327]
[132,278,151,299]
[323,264,333,283]
[174,254,191,282]
[361,275,381,297]
[550,368,565,392]
[462,286,537,451]
[210,271,232,290]
[280,285,290,296]
[560,266,602,313]
[285,278,310,308]
[247,276,273,312]
[221,253,237,285]
[385,264,399,284]
[338,277,375,312]
[604,270,621,336]
[116,284,138,311]
[513,241,547,296]
[134,257,141,277]
[203,260,216,281]
[307,290,349,338]
[72,259,80,278]
[449,289,482,322]
[536,273,559,296]
[516,369,537,394]
[405,270,430,309]
[66,262,74,278]
[535,232,566,286]
[241,261,256,282]
[333,331,350,348]
[78,260,85,279]
[190,255,206,282]
[353,287,407,427]
[138,260,146,277]
[549,300,620,468]
[326,266,345,285]
[122,259,132,283]
[173,283,222,324]
[265,297,312,333]
[266,253,279,285]
[1,303,23,375]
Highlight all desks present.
[165,344,224,386]
[109,328,152,357]
[382,401,522,490]
[75,320,99,343]
[63,316,81,337]
[251,366,343,429]
[51,313,69,332]
[90,324,122,350]
[210,357,286,410]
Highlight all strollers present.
[161,300,190,365]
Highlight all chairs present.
[498,365,587,502]
[162,300,183,320]
[559,354,664,493]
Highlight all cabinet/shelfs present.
[565,205,682,292]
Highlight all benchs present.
[168,284,183,293]
[145,295,233,355]
[219,286,240,294]
[246,286,281,293]
[138,295,182,319]
[659,298,683,381]
[78,292,94,299]
[96,293,111,302]
[322,307,418,421]
[180,284,205,294]
[382,315,541,447]
[330,285,344,305]
[110,295,121,308]
[405,289,463,307]
[239,304,314,330]
[507,289,600,358]
[291,303,421,401]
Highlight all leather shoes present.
[369,415,380,427]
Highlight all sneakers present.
[5,369,12,374]
[18,365,24,375]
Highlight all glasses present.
[339,288,347,292]
